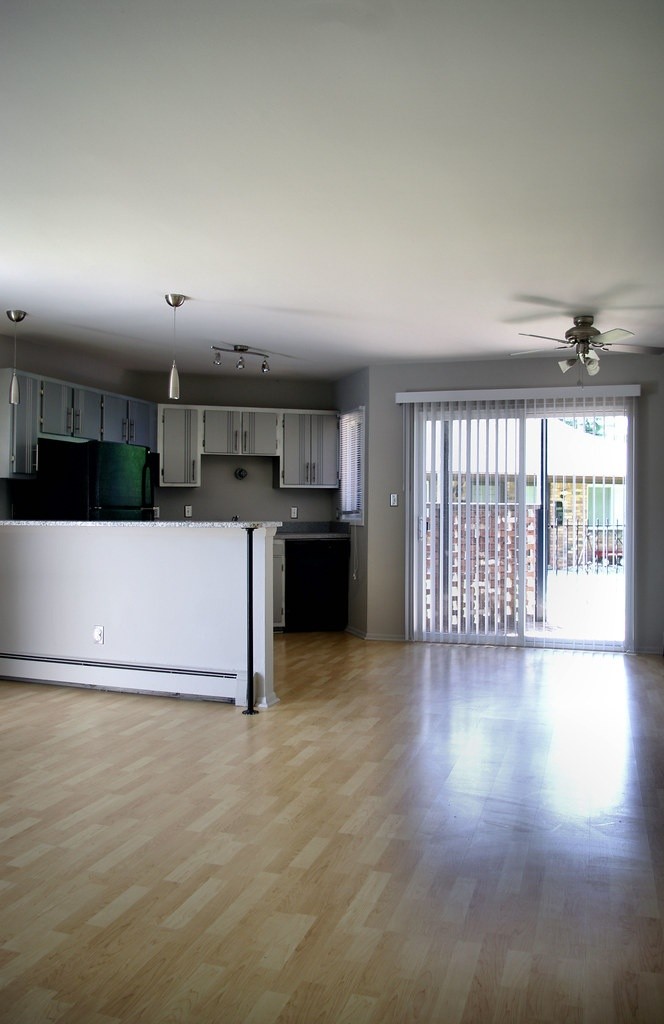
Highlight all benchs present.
[594,550,624,566]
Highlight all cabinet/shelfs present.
[156,403,202,487]
[0,368,42,481]
[272,406,344,490]
[38,374,158,453]
[272,539,286,634]
[201,406,282,457]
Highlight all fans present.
[508,316,664,358]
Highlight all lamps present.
[6,309,28,405]
[557,343,601,391]
[165,294,186,399]
[210,345,271,373]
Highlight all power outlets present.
[290,507,298,518]
[185,505,193,517]
[153,507,159,519]
[391,494,398,506]
